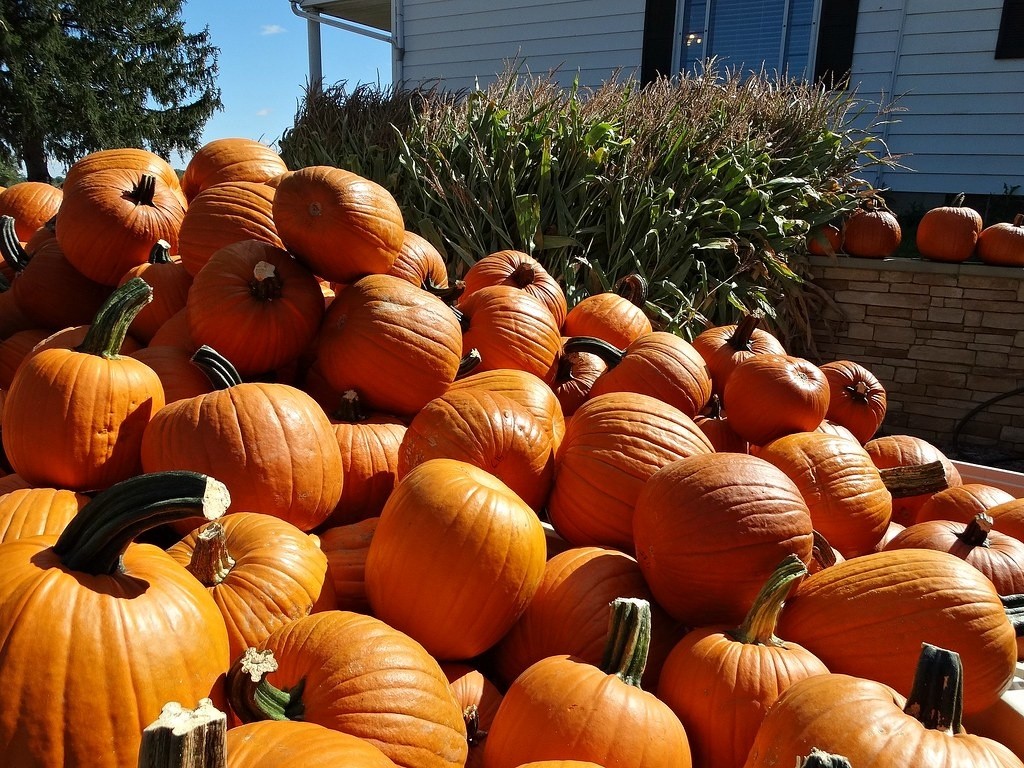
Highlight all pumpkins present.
[0,139,1024,768]
